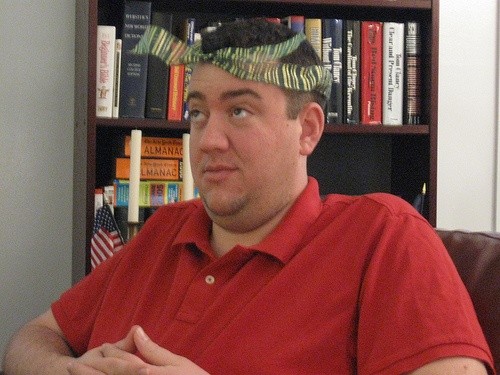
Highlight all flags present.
[90,205,128,268]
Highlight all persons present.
[3,19,496,375]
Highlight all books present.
[95,135,199,213]
[95,0,421,126]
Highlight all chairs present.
[432,228,500,375]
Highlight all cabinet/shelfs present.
[71,0,439,287]
